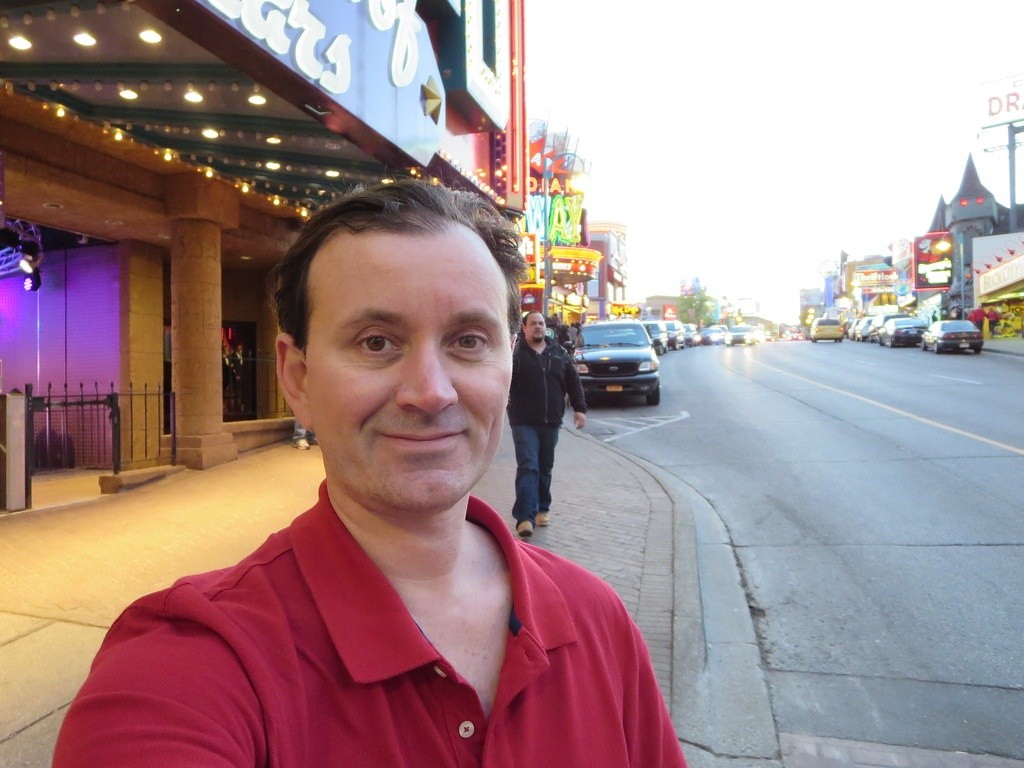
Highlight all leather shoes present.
[516,521,533,537]
[535,511,551,525]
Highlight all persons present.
[956,304,999,336]
[51,180,687,768]
[292,419,318,450]
[506,311,587,537]
[545,313,581,353]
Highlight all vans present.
[700,328,725,343]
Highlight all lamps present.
[19,240,41,291]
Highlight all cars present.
[922,320,984,353]
[810,319,843,343]
[571,319,660,408]
[641,321,668,356]
[844,322,851,339]
[868,315,911,343]
[765,336,776,342]
[848,319,861,341]
[749,328,765,344]
[879,318,927,347]
[707,325,728,332]
[684,325,700,347]
[855,318,873,342]
[727,326,750,345]
[663,321,686,350]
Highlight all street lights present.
[542,153,587,318]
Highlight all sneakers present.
[292,439,310,449]
